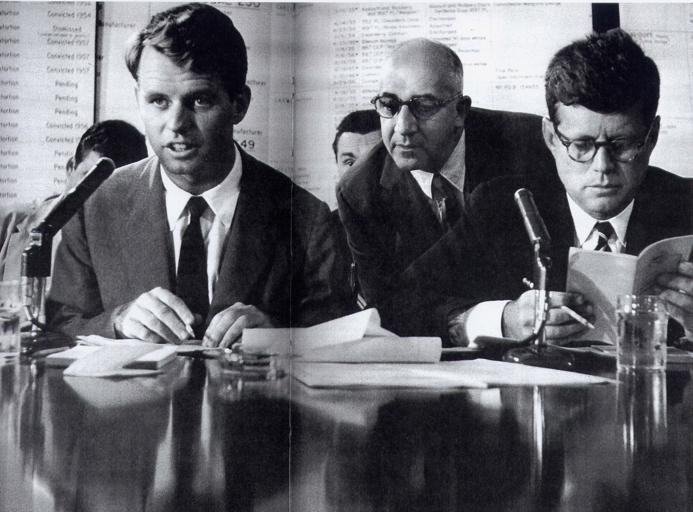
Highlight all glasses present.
[551,116,656,163]
[371,92,462,121]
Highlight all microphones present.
[503,188,554,267]
[31,157,116,241]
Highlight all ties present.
[594,223,612,252]
[173,196,209,339]
[430,173,460,232]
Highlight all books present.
[277,358,613,392]
[561,233,693,347]
[44,331,180,370]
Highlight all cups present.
[0,279,33,354]
[616,295,668,367]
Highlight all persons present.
[328,35,553,324]
[327,107,394,208]
[1,116,151,341]
[358,27,692,357]
[28,2,347,344]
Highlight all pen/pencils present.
[522,278,597,330]
[183,320,198,338]
